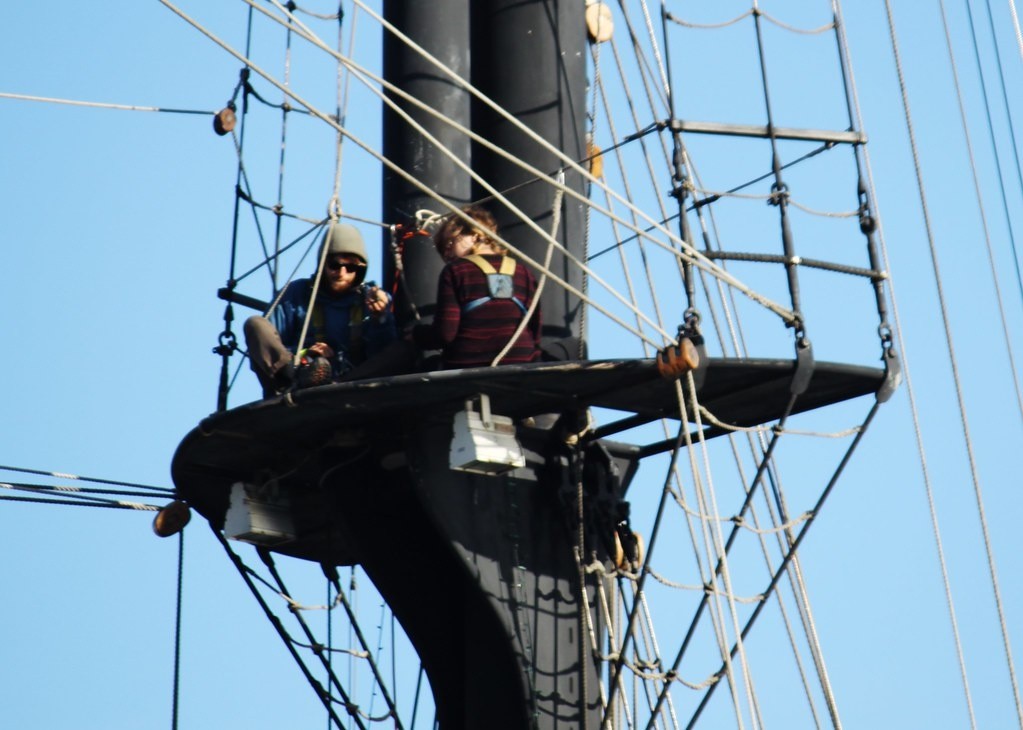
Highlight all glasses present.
[442,232,465,253]
[324,260,361,273]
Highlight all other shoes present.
[273,358,331,392]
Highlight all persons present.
[244,224,397,395]
[403,208,588,462]
